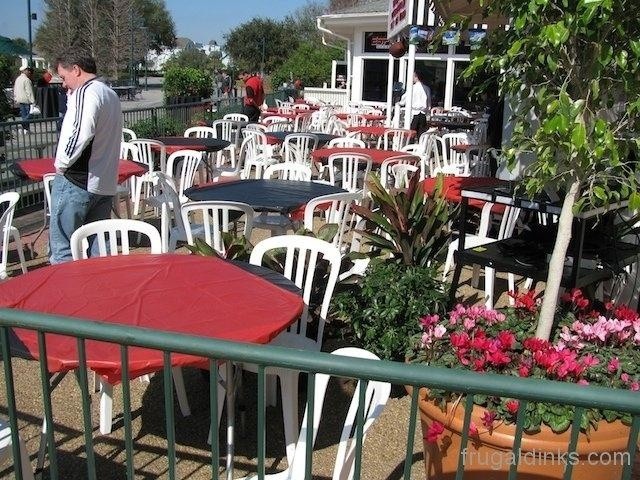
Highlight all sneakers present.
[18,128,31,135]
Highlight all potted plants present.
[404,0,640,230]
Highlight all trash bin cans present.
[392,88,402,106]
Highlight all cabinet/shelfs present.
[447,180,640,318]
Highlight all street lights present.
[129,9,148,98]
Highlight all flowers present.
[403,286,640,446]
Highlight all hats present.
[19,66,31,71]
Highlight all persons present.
[213,69,260,106]
[48,47,122,266]
[401,71,432,151]
[13,66,36,135]
[282,76,302,99]
[42,69,52,88]
[27,69,35,87]
[240,70,265,138]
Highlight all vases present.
[404,380,639,480]
